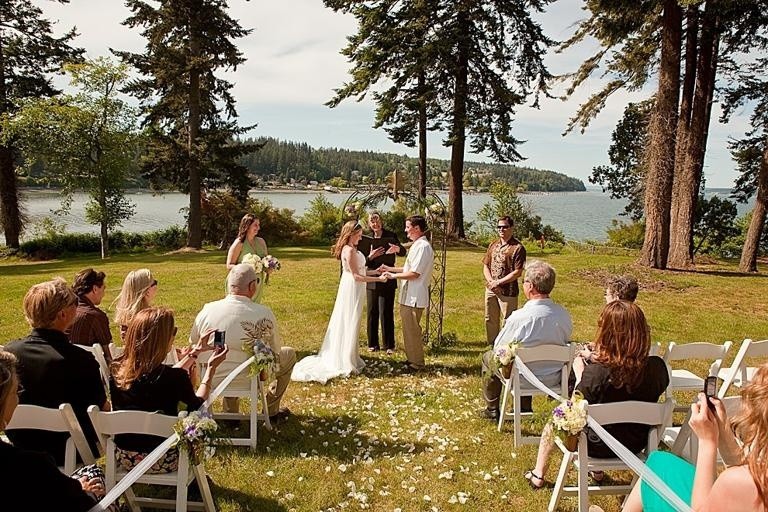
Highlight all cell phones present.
[214,330,225,349]
[704,376,716,411]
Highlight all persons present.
[226,211,269,305]
[577,272,640,362]
[588,361,768,512]
[316,220,388,377]
[354,211,407,356]
[186,262,298,433]
[1,266,228,511]
[380,214,435,375]
[522,299,671,490]
[475,258,573,421]
[480,216,527,345]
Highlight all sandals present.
[589,471,604,483]
[523,471,544,489]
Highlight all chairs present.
[4,403,95,478]
[497,342,576,447]
[547,398,675,512]
[650,339,768,465]
[108,342,179,370]
[87,405,215,511]
[194,348,273,453]
[73,342,110,391]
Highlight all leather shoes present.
[479,407,499,422]
[277,407,290,419]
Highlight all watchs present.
[496,280,500,286]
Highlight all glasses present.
[248,278,260,291]
[499,223,513,229]
[151,280,157,287]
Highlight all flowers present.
[256,350,274,368]
[553,396,588,435]
[242,253,262,275]
[500,339,521,366]
[178,408,218,440]
[262,255,281,283]
